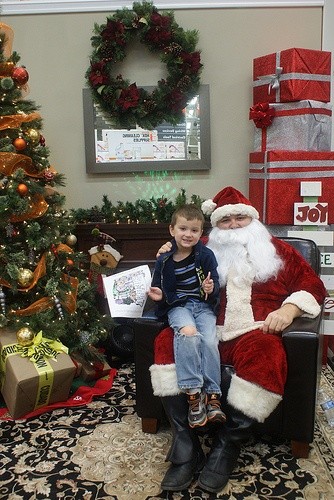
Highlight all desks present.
[74,222,213,272]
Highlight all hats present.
[201,185,259,227]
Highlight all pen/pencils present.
[204,271,211,300]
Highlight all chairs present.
[134,237,323,458]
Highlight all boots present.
[197,403,258,493]
[160,393,204,490]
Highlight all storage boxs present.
[0,326,113,419]
[248,48,334,227]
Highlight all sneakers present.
[186,390,207,428]
[204,394,227,424]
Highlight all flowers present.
[85,0,205,129]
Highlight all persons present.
[156,186,327,492]
[146,204,226,429]
